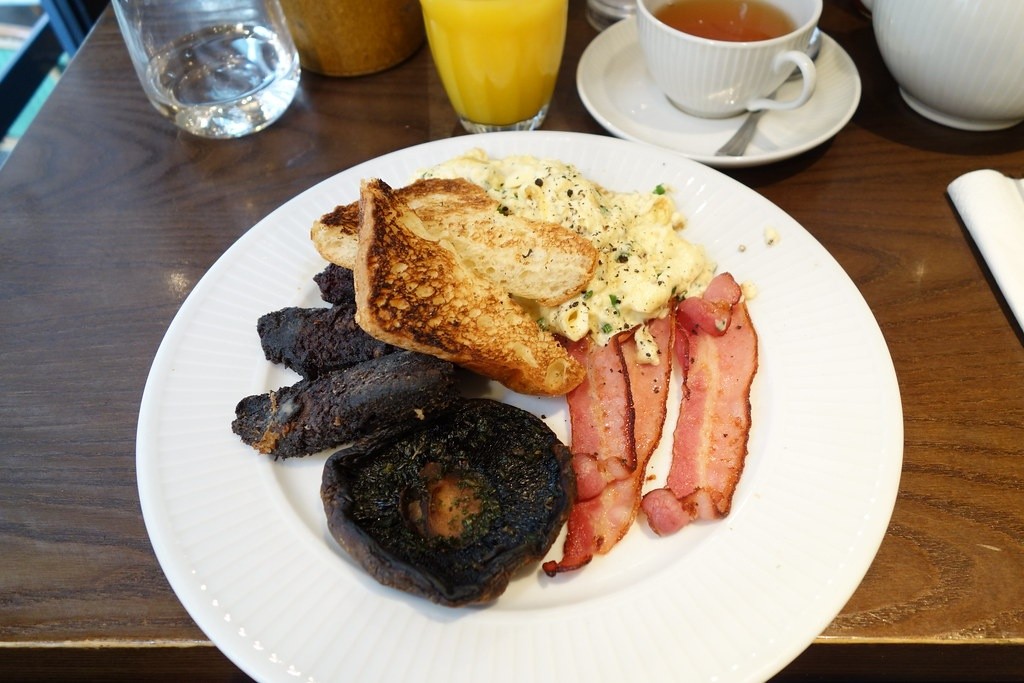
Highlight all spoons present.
[715,27,822,159]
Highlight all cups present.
[872,0,1024,131]
[635,0,823,120]
[111,0,301,139]
[263,0,417,77]
[419,0,568,134]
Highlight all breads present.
[312,176,597,396]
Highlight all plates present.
[577,17,860,166]
[135,130,904,682]
